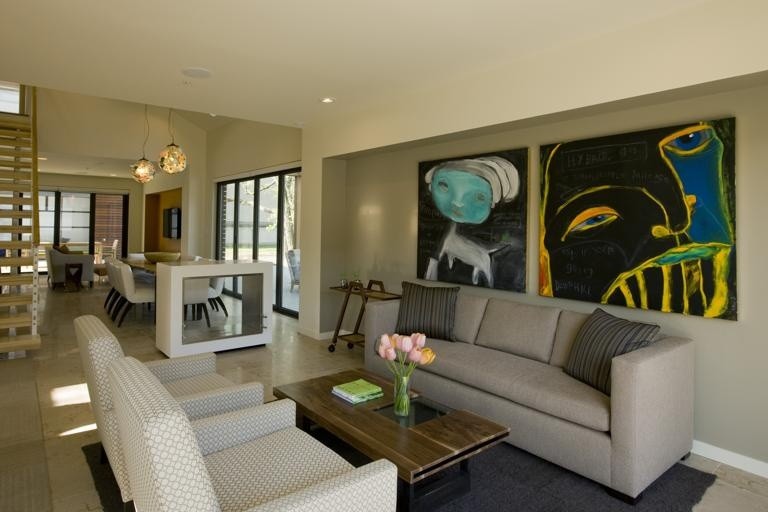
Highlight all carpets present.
[78,394,718,512]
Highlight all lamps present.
[128,102,159,184]
[154,104,188,175]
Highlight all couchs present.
[362,292,701,505]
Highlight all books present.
[330,389,384,402]
[331,390,354,404]
[333,377,382,397]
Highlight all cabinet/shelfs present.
[153,259,277,358]
[324,276,402,357]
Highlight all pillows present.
[564,307,661,397]
[395,280,460,343]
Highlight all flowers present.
[370,326,436,414]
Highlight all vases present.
[391,374,412,417]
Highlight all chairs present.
[73,314,267,505]
[285,249,305,294]
[45,244,232,329]
[99,353,400,511]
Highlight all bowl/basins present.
[143,251,180,264]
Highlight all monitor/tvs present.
[163,208,180,239]
